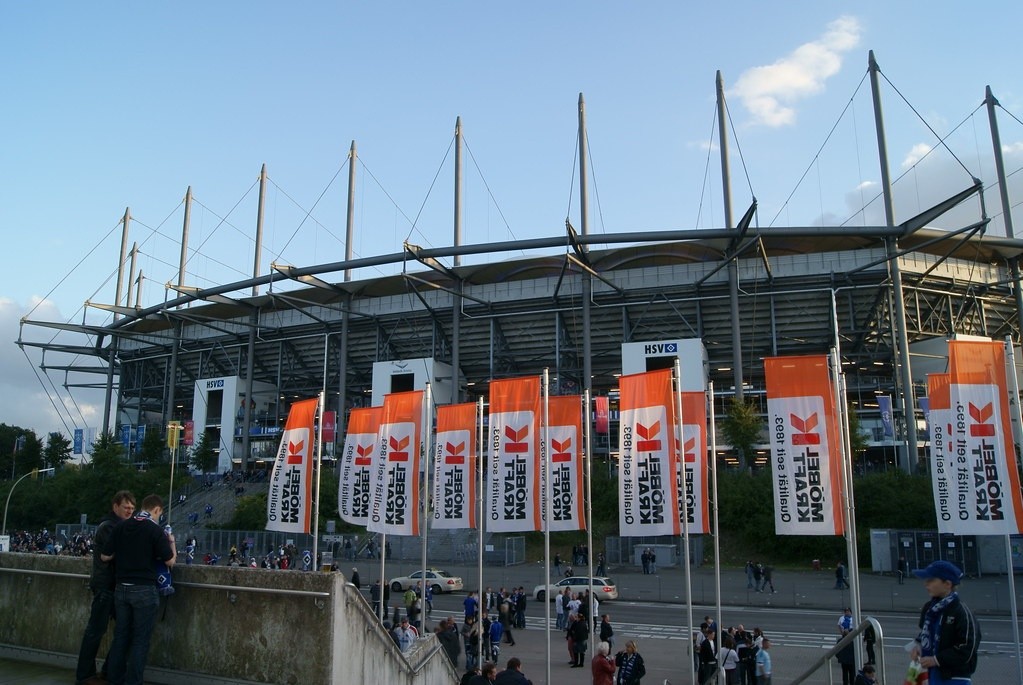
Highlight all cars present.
[388,569,464,594]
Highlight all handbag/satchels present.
[904,656,930,685]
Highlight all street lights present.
[2,467,56,535]
[166,422,186,526]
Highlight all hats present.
[400,616,408,623]
[383,620,392,629]
[912,560,963,586]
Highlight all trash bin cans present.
[572,546,588,567]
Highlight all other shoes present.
[511,641,515,646]
[82,677,109,685]
[485,660,489,663]
[864,661,876,665]
[571,663,584,668]
[568,661,574,664]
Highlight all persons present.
[184,503,321,571]
[836,563,849,589]
[74,492,176,685]
[179,469,268,506]
[744,560,774,592]
[554,544,607,578]
[556,589,646,685]
[687,616,772,685]
[641,550,656,574]
[911,561,981,685]
[370,580,432,652]
[328,539,391,590]
[898,556,905,584]
[863,625,876,665]
[835,608,856,685]
[434,587,532,685]
[856,666,879,685]
[5,527,95,557]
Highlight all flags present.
[265,339,1023,536]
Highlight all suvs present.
[532,575,618,603]
